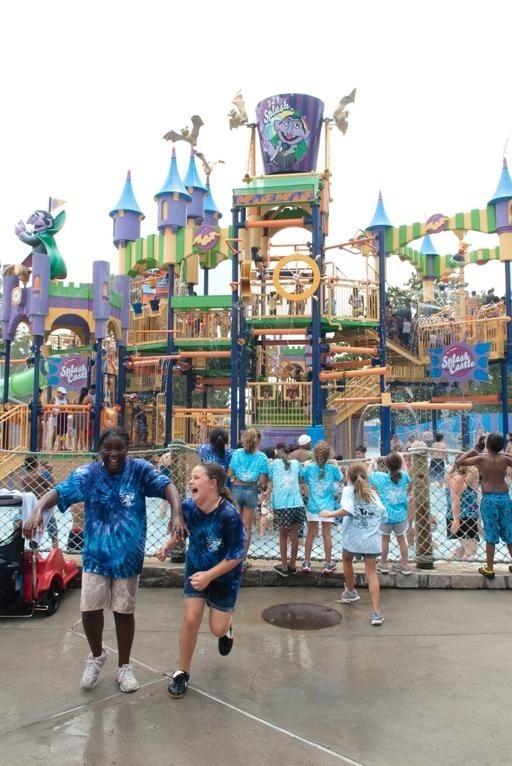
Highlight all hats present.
[298,435,311,445]
[57,386,67,393]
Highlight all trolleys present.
[0,491,79,617]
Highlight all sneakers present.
[274,560,337,577]
[219,616,233,655]
[241,560,253,572]
[81,648,107,690]
[376,563,389,573]
[371,611,384,625]
[116,664,139,692]
[391,564,411,574]
[336,590,360,602]
[478,567,495,579]
[168,670,190,699]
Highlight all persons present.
[158,439,188,521]
[390,428,450,551]
[186,310,231,338]
[455,433,512,577]
[66,502,84,550]
[286,366,312,399]
[346,287,506,355]
[156,462,247,699]
[197,427,387,577]
[444,431,512,560]
[25,357,35,369]
[319,462,388,625]
[24,425,193,694]
[28,384,148,451]
[267,281,335,316]
[21,455,58,549]
